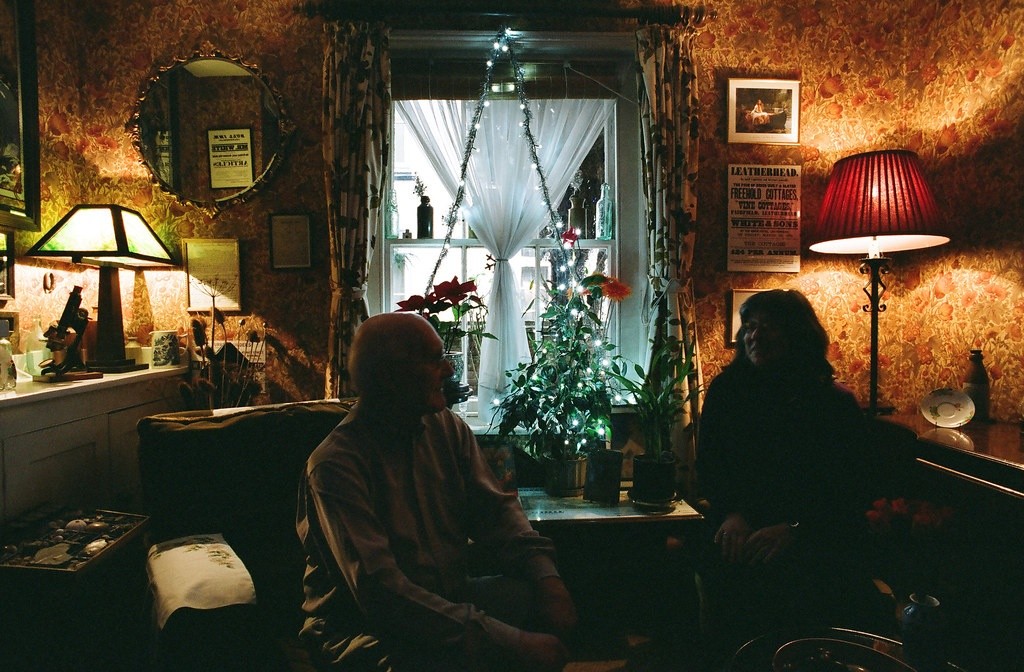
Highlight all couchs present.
[136,399,356,672]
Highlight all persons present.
[691,290,867,630]
[752,99,769,124]
[294,312,577,672]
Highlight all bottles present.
[417,196,433,239]
[962,350,989,424]
[901,592,943,664]
[26,314,51,376]
[568,197,587,239]
[595,183,612,240]
[0,336,17,392]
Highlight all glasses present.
[381,353,446,366]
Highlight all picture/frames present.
[726,77,802,147]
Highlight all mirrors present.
[123,39,298,220]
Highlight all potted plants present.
[601,279,709,502]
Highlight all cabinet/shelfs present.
[0,364,193,529]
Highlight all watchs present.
[788,519,800,528]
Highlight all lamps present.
[26,204,180,374]
[808,149,950,416]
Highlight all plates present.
[919,388,976,428]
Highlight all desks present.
[867,412,1024,500]
[516,488,704,527]
[731,625,969,672]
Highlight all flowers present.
[481,224,630,459]
[394,275,501,354]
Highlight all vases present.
[443,349,464,383]
[543,452,587,496]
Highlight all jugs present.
[148,330,189,369]
